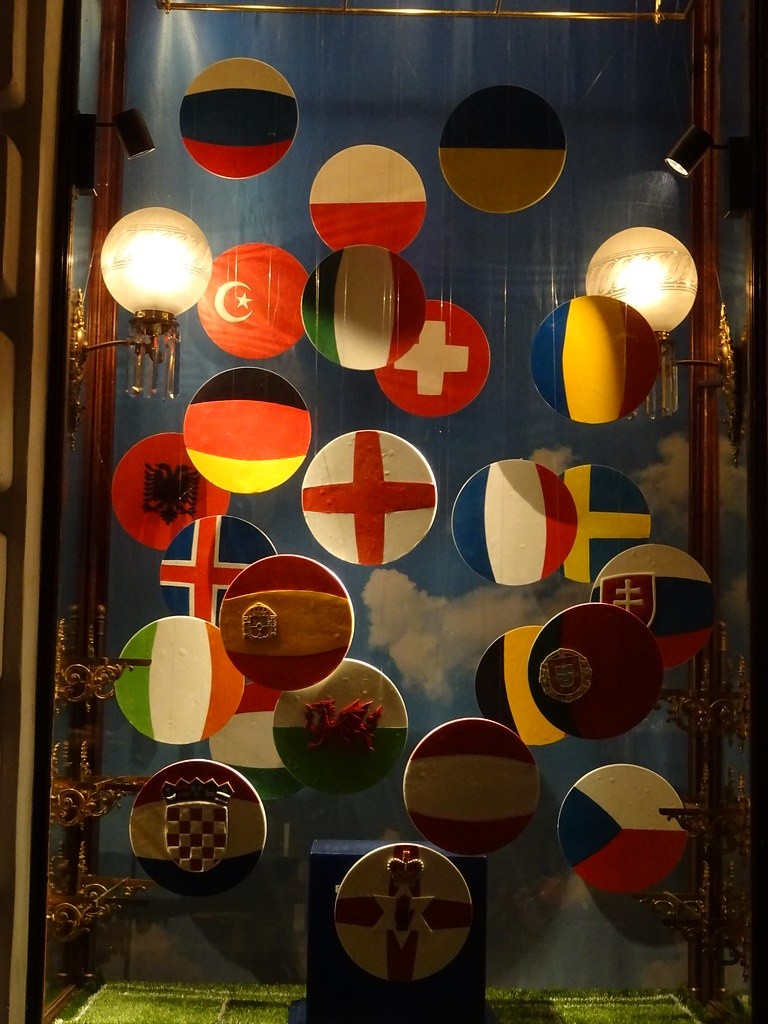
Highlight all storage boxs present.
[284,839,500,1024]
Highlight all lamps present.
[586,226,745,472]
[65,208,214,455]
[666,124,756,221]
[74,107,157,198]
